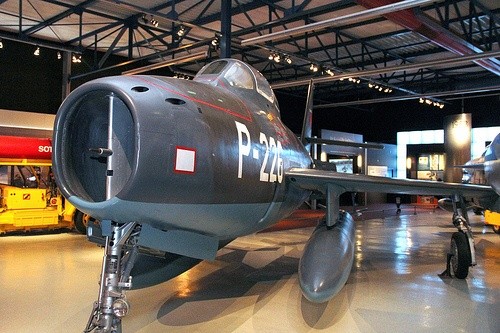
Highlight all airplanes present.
[48,56,500,333]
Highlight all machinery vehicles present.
[0,135,94,235]
